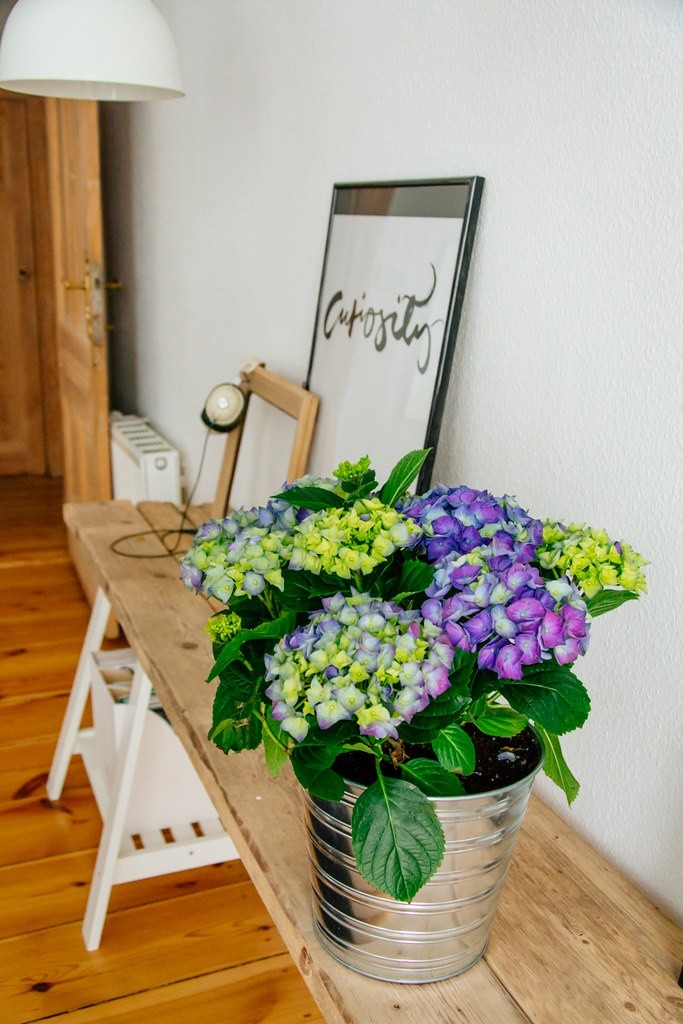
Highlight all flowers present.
[177,447,651,902]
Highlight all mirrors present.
[212,365,320,522]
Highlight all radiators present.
[109,411,182,504]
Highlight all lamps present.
[0,0,185,102]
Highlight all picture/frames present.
[300,176,484,494]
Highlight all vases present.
[302,714,548,984]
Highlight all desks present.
[44,500,683,1024]
